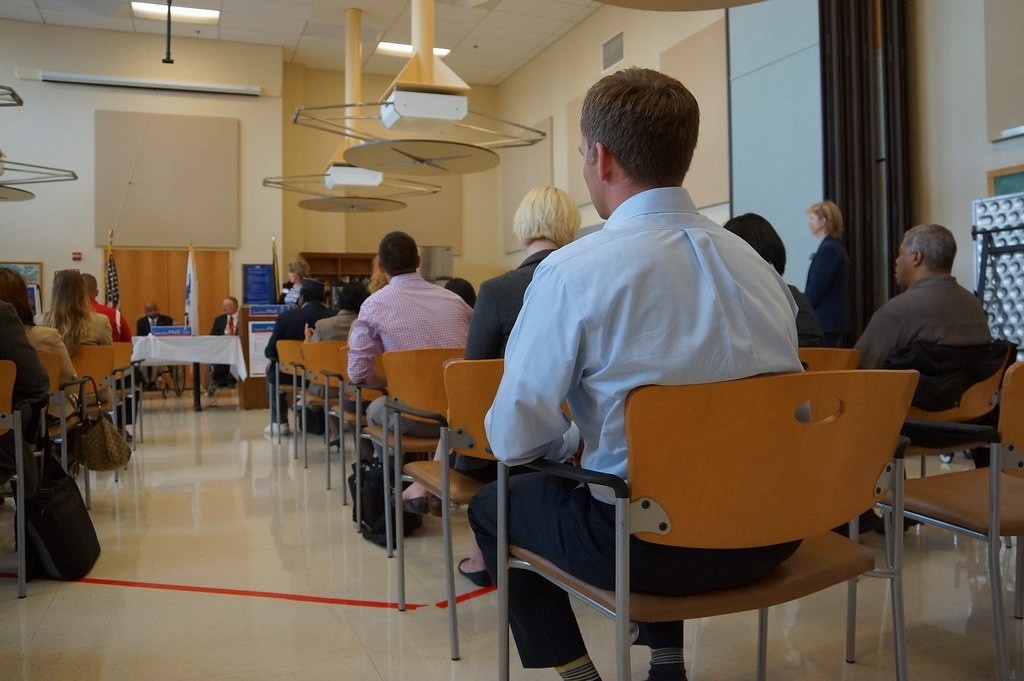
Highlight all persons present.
[722,212,824,348]
[205,296,239,398]
[803,200,851,348]
[367,268,389,295]
[466,66,807,681]
[291,281,371,454]
[390,186,582,587]
[137,302,174,391]
[444,277,477,310]
[0,268,144,551]
[264,281,341,436]
[277,259,327,306]
[346,230,474,517]
[854,223,991,534]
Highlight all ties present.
[229,316,233,335]
[151,320,155,326]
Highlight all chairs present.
[274,340,1024,681]
[0,342,143,598]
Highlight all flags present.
[184,245,200,335]
[268,242,281,306]
[104,239,120,310]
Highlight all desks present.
[131,335,239,411]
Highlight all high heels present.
[329,439,340,452]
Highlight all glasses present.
[54,269,80,277]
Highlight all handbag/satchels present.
[299,407,325,434]
[78,376,131,471]
[12,460,100,579]
[348,460,423,548]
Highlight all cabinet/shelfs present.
[300,252,377,308]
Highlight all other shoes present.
[875,509,918,534]
[430,496,442,516]
[265,422,289,434]
[459,558,486,586]
[404,497,429,514]
[118,427,132,442]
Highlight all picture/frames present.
[0,262,44,314]
